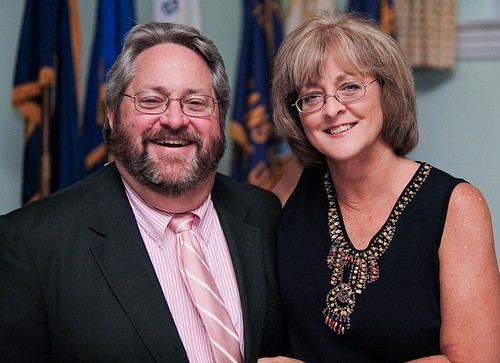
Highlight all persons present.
[0,21,283,363]
[257,9,500,363]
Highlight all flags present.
[154,0,201,38]
[12,0,81,207]
[84,0,138,179]
[229,0,396,204]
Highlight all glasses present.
[291,77,377,113]
[120,91,221,117]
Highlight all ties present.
[168,212,243,363]
